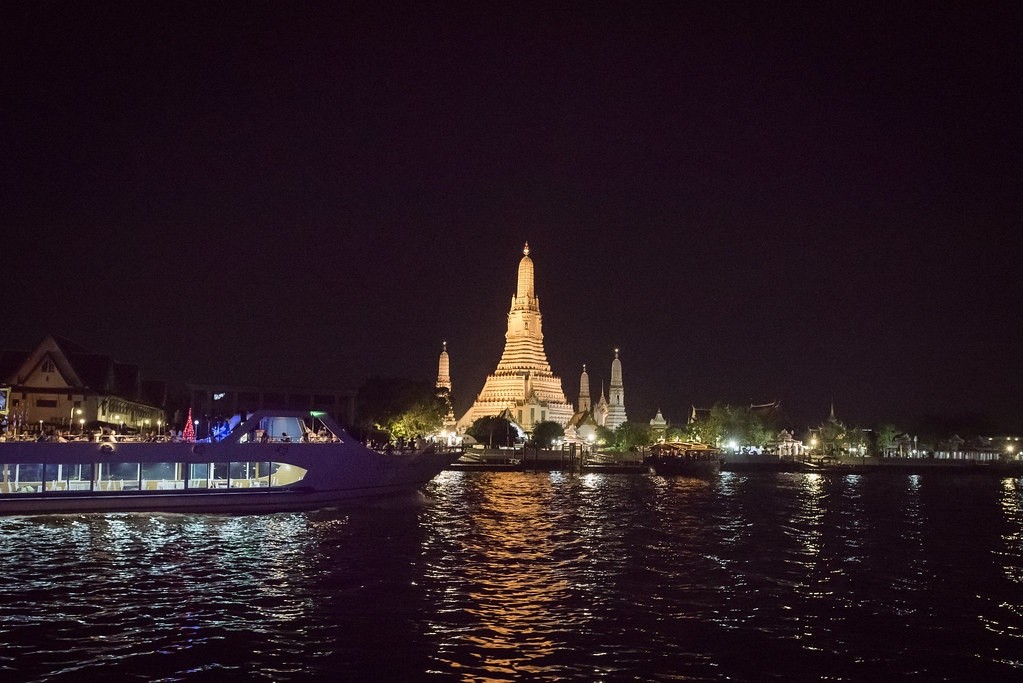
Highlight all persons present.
[280,432,290,442]
[317,425,327,436]
[300,432,308,443]
[359,433,471,453]
[261,430,269,445]
[164,425,177,442]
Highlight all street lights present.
[69,408,81,434]
[194,420,199,438]
[157,417,161,437]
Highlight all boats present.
[0,407,467,512]
[645,441,722,478]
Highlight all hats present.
[302,432,308,436]
